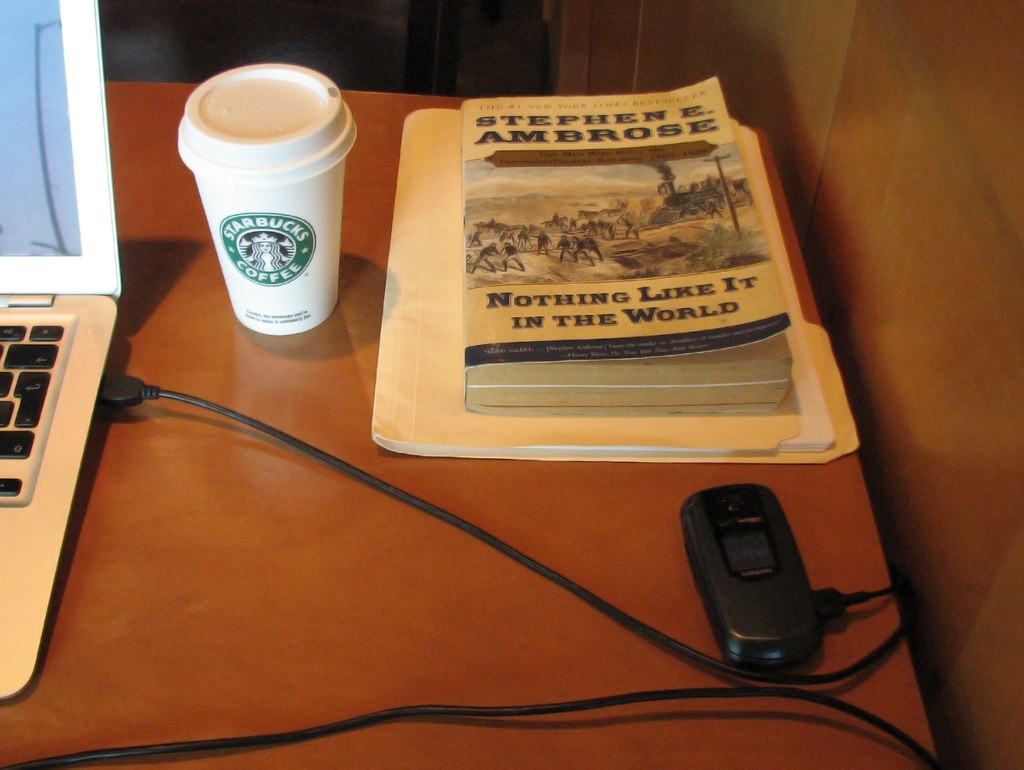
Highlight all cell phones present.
[680,483,825,675]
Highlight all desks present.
[0,82,943,770]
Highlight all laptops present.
[0,0,122,699]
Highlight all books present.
[370,74,862,466]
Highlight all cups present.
[177,64,358,337]
